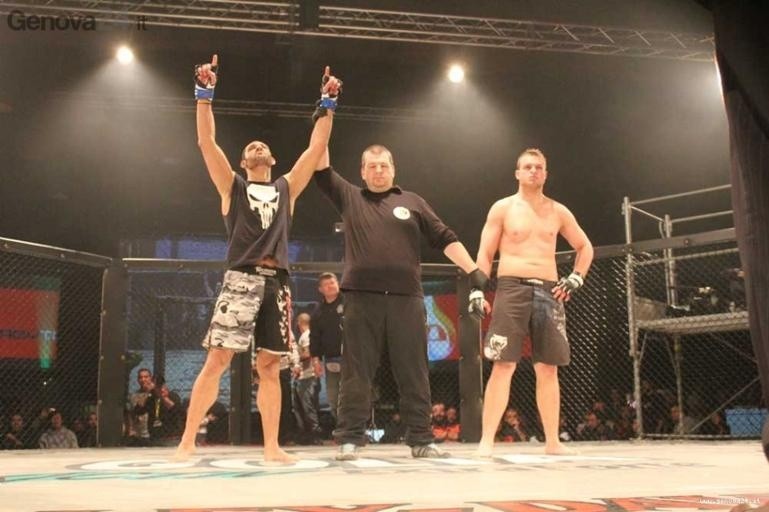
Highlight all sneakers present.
[412,444,450,458]
[336,444,360,460]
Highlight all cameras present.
[150,387,162,398]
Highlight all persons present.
[310,97,490,459]
[171,52,344,467]
[378,378,742,441]
[276,330,301,450]
[467,147,594,456]
[1,366,227,449]
[289,313,325,445]
[310,273,347,447]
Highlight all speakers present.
[299,0,320,29]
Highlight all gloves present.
[311,77,344,125]
[557,271,584,295]
[468,269,489,321]
[194,63,218,101]
